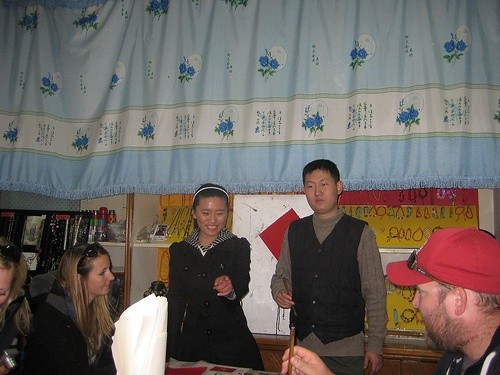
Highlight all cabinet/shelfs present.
[79,194,234,320]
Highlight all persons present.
[270,159,388,375]
[164,183,266,375]
[281,227,500,375]
[28,244,116,375]
[0,236,34,375]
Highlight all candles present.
[395,310,398,322]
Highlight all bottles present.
[79,207,117,241]
[0,347,18,369]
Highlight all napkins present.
[113,291,167,374]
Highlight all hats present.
[386,226,500,295]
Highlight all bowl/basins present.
[107,223,126,243]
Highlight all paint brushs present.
[281,275,298,317]
[287,321,296,375]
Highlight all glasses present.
[81,242,104,257]
[407,248,451,290]
[0,244,21,262]
